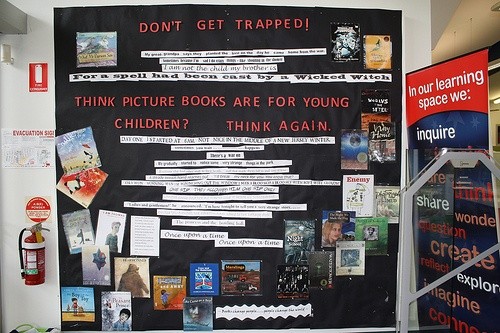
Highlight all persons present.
[321,221,344,247]
[364,227,379,240]
[65,220,208,330]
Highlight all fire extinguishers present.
[18,223,52,286]
